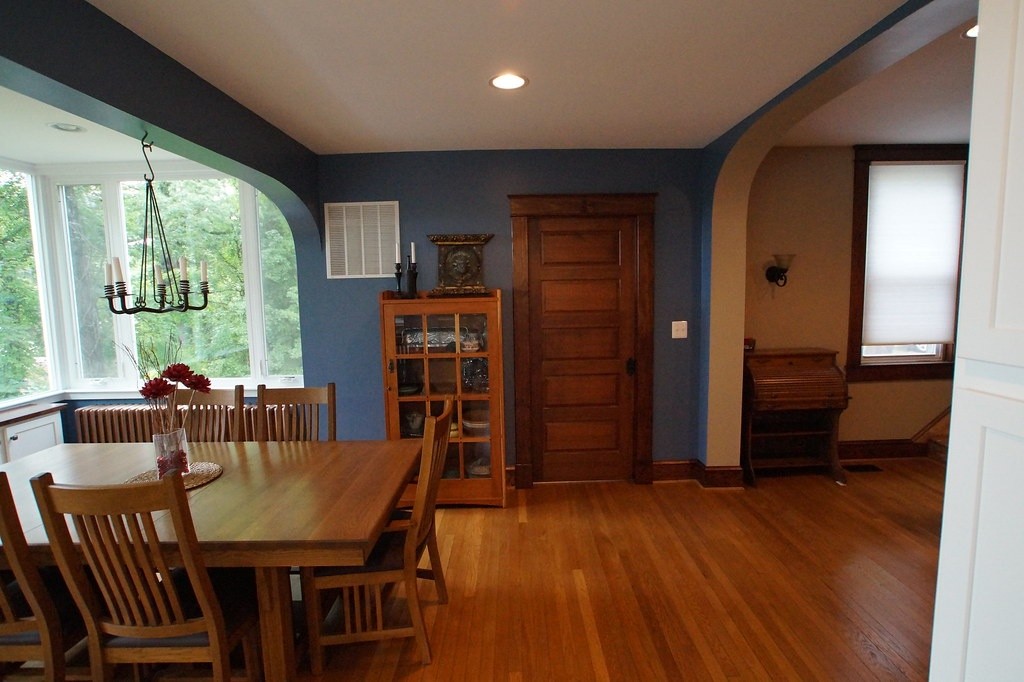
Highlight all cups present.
[406,414,423,429]
[464,340,478,348]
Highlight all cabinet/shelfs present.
[380,287,508,509]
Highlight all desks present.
[0,437,424,682]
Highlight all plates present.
[462,409,490,428]
[471,466,490,476]
[399,387,418,393]
[461,347,479,352]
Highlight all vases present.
[153,427,191,480]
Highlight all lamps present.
[766,252,797,286]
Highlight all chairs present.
[0,380,460,682]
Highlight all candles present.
[104,253,209,296]
[395,240,416,263]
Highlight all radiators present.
[74,402,309,443]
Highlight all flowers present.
[129,335,214,432]
